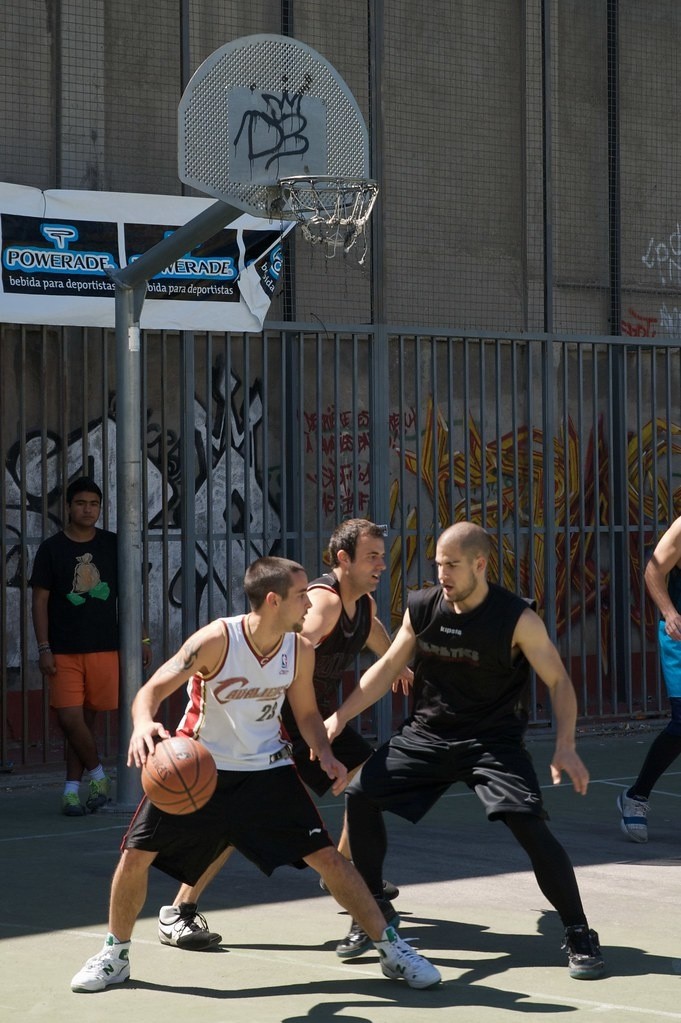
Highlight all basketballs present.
[144,733,215,815]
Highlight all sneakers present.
[158,901,223,951]
[71,932,132,993]
[319,861,399,900]
[335,895,400,958]
[371,926,441,987]
[61,791,86,816]
[85,773,112,809]
[617,787,650,843]
[560,924,605,979]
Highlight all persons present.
[309,522,605,980]
[28,476,153,817]
[69,517,442,993]
[616,514,681,843]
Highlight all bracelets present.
[142,637,151,646]
[37,640,52,656]
